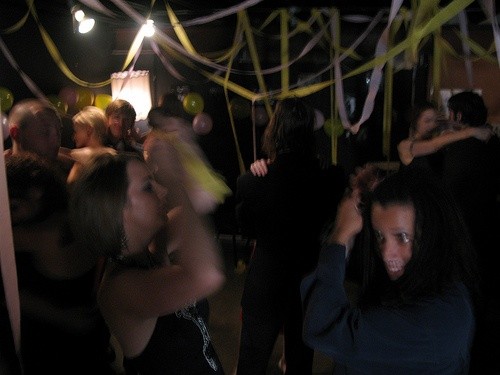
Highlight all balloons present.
[0,47,386,141]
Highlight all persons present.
[0,93,500,375]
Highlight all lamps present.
[71,6,96,34]
